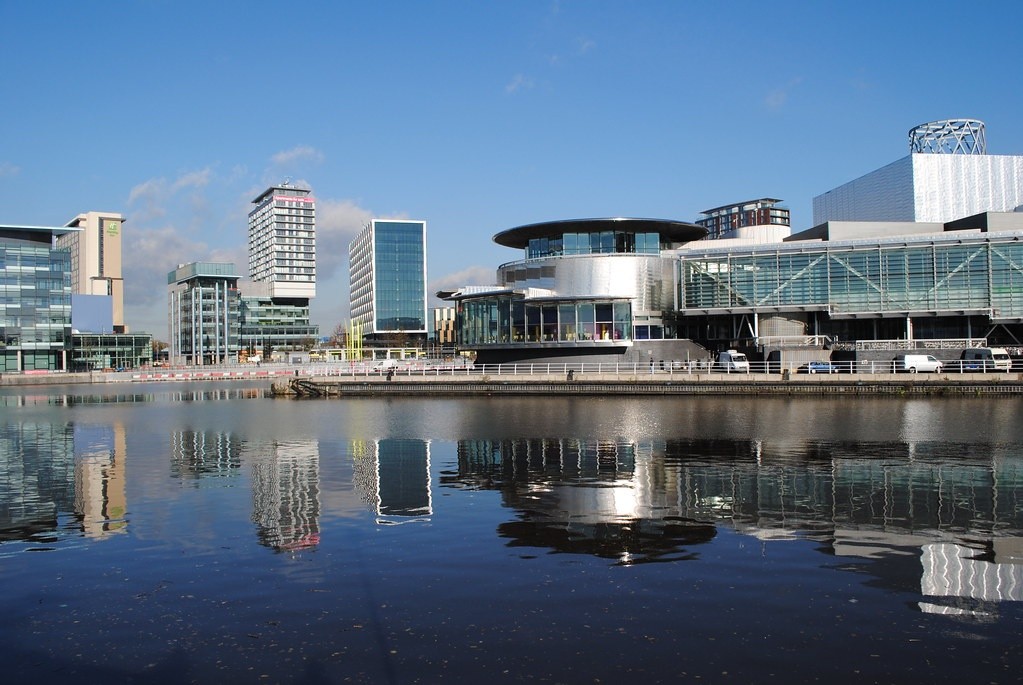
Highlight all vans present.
[896,353,944,374]
[965,347,1013,370]
[719,351,750,372]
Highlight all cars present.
[802,361,842,374]
